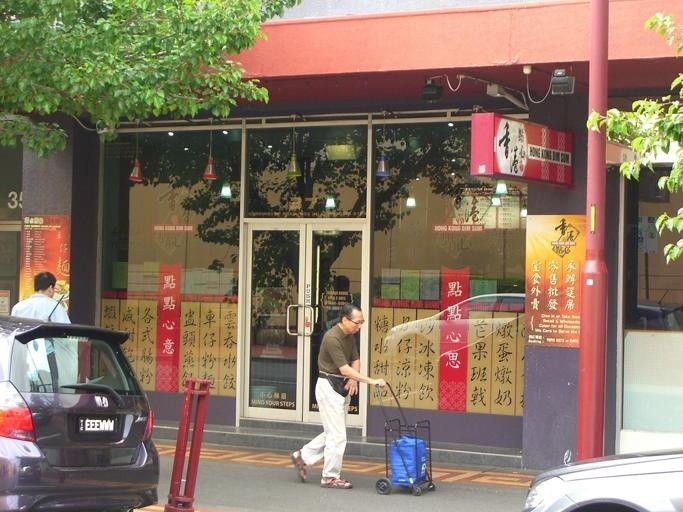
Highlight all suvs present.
[0,310,162,512]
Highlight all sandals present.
[320,477,353,490]
[292,450,306,481]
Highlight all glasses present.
[346,318,364,325]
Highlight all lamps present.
[375,107,392,178]
[547,68,575,99]
[323,192,335,209]
[284,112,304,178]
[494,176,507,195]
[406,187,415,208]
[418,78,444,105]
[220,179,231,199]
[128,118,150,185]
[202,114,218,184]
[490,193,502,207]
[518,197,527,218]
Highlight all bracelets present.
[374,379,380,387]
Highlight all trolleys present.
[374,376,438,498]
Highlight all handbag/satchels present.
[390,436,426,484]
[326,376,351,396]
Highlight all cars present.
[522,449,682,512]
[432,313,524,383]
[634,296,683,331]
[379,286,526,383]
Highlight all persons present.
[309,276,360,325]
[12,271,73,393]
[290,303,386,489]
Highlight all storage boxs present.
[378,261,524,304]
[111,256,239,297]
